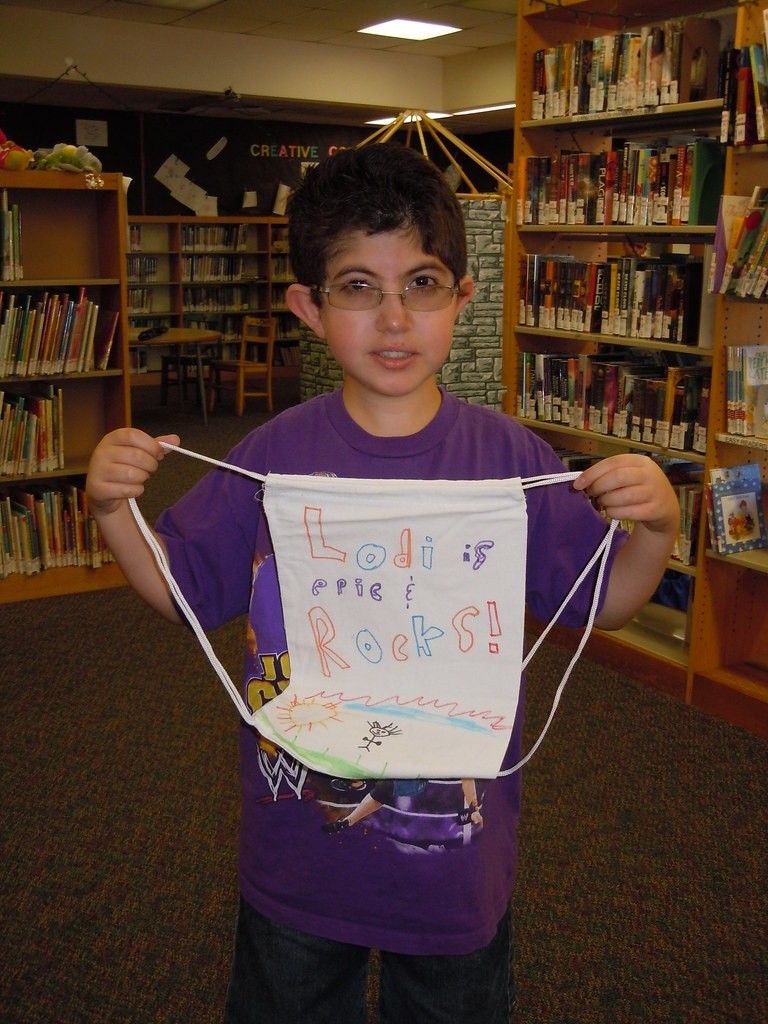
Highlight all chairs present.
[209,316,277,417]
[160,316,222,408]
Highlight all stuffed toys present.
[0,131,103,175]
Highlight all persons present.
[86,144,680,1024]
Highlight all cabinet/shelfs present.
[0,172,298,605]
[502,0,768,741]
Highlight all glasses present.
[308,282,462,311]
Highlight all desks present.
[128,327,222,428]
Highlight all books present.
[0,191,117,577]
[127,221,301,375]
[513,15,768,565]
